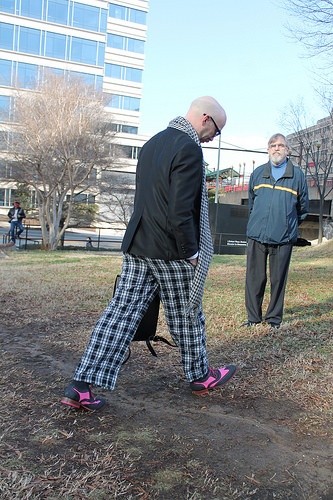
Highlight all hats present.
[12,200,20,205]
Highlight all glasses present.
[204,113,221,136]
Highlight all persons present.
[86,237,92,247]
[60,96,237,412]
[240,133,310,329]
[6,201,26,243]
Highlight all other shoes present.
[15,235,20,239]
[239,323,259,329]
[11,237,15,242]
[266,323,279,330]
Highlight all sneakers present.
[190,366,238,394]
[61,386,108,414]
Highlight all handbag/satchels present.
[113,275,179,365]
